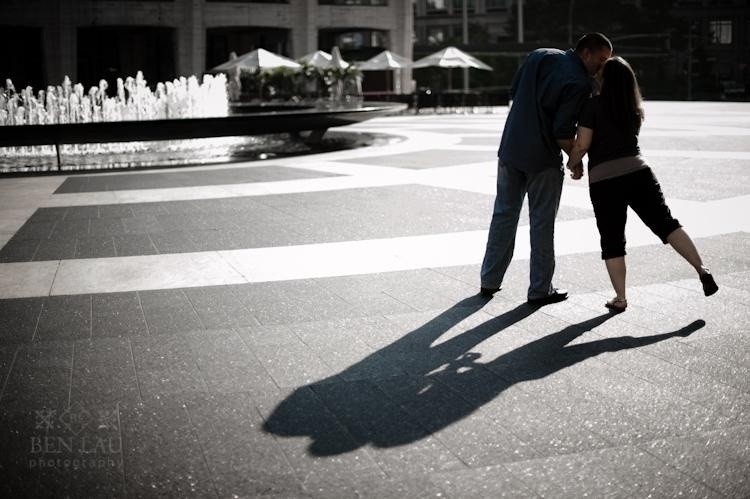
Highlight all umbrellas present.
[213,48,304,73]
[355,50,414,91]
[295,50,350,70]
[408,46,495,90]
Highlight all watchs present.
[566,162,571,171]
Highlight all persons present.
[566,56,719,310]
[480,31,613,305]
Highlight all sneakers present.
[607,297,627,310]
[699,271,718,296]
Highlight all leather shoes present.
[481,287,499,296]
[528,289,568,304]
